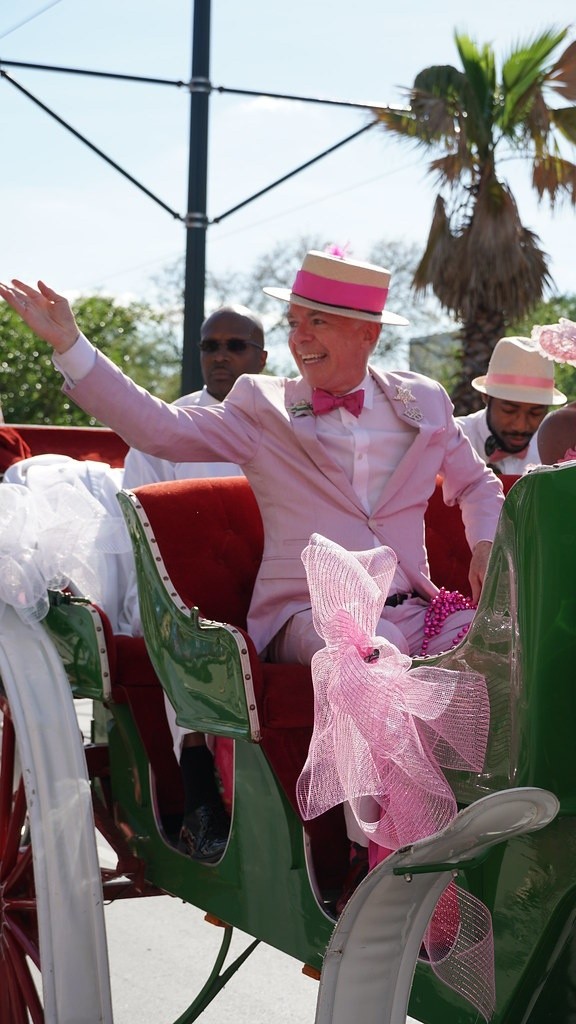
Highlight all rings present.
[22,301,25,305]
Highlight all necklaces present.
[420,586,478,659]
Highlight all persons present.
[0,250,504,887]
[453,335,568,475]
[122,304,268,490]
[526,318,576,474]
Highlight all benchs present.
[0,424,576,903]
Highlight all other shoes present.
[336,859,369,912]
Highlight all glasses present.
[196,339,263,351]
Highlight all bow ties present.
[312,387,364,418]
[488,445,529,464]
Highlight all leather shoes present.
[383,591,420,608]
[177,801,230,863]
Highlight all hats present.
[262,250,409,326]
[471,336,568,405]
